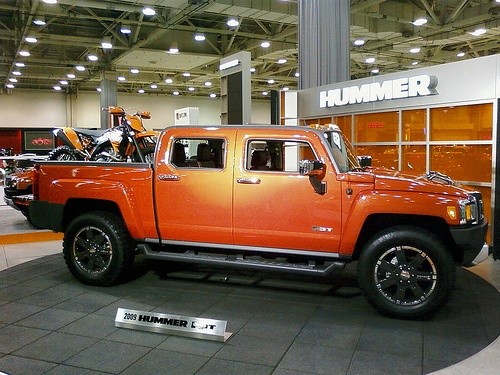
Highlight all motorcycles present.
[47,106,156,163]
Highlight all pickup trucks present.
[4,125,489,319]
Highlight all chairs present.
[173,143,189,167]
[194,143,216,168]
[252,150,270,170]
